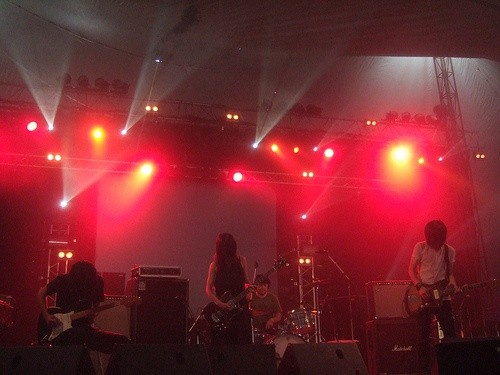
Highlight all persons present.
[408,219,460,375]
[205,232,257,346]
[37,261,131,375]
[247,273,283,345]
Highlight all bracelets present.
[269,318,275,323]
[414,282,423,291]
[215,300,220,306]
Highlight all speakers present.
[208,342,280,375]
[129,276,187,345]
[0,345,96,375]
[367,318,438,375]
[105,344,211,375]
[277,340,366,375]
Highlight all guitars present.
[402,272,498,319]
[202,253,287,334]
[45,296,144,343]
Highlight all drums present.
[283,306,315,337]
